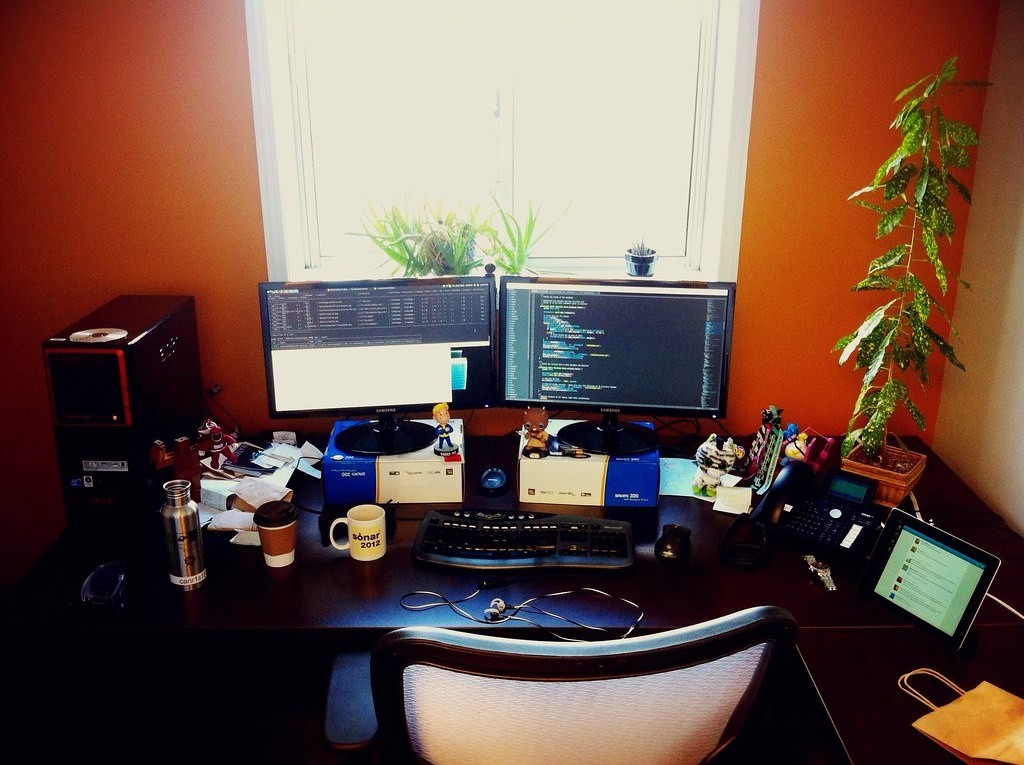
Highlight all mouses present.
[655,523,692,563]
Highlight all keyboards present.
[414,509,634,570]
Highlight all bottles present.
[160,480,206,591]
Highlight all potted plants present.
[354,194,567,274]
[624,237,660,276]
[838,54,999,509]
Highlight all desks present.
[0,439,1023,764]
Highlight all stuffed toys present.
[691,433,738,498]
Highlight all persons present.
[199,426,238,468]
[431,401,454,449]
[522,405,551,459]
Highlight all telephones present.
[748,460,894,587]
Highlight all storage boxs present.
[514,418,661,509]
[320,416,469,504]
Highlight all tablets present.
[857,507,1001,651]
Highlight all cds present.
[69,327,128,343]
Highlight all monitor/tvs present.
[259,274,498,456]
[497,276,736,456]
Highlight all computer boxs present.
[43,294,210,565]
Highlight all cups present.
[253,500,297,567]
[329,503,386,560]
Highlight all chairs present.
[315,601,799,765]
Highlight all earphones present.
[489,609,513,620]
[497,601,515,612]
